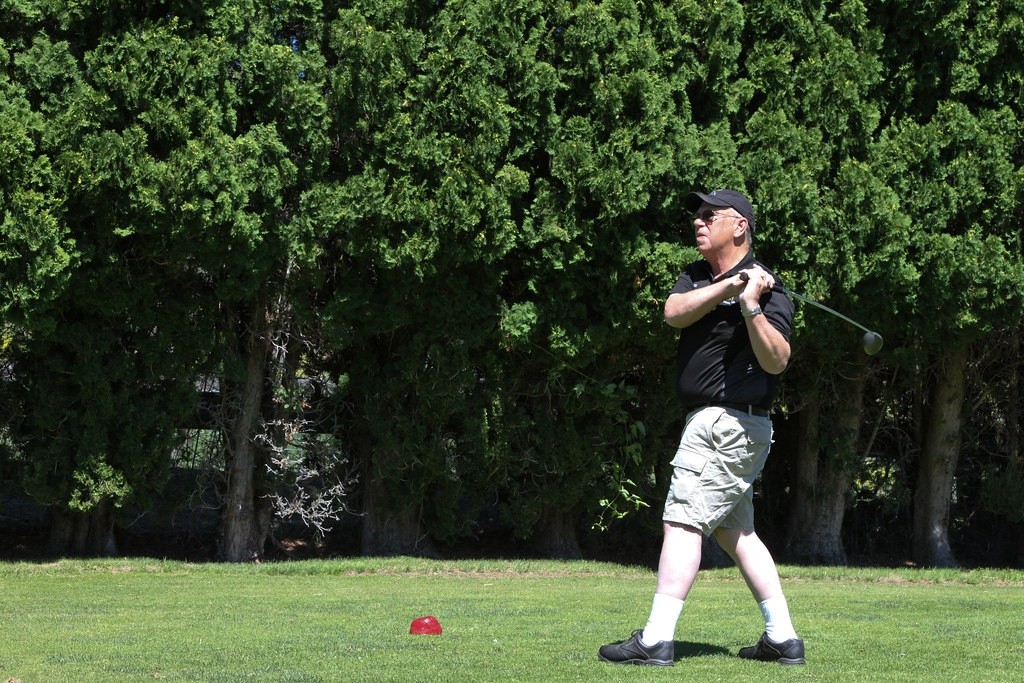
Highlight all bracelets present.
[741,303,762,318]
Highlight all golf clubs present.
[739,272,884,356]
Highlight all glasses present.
[687,209,742,224]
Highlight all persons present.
[598,190,807,668]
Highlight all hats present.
[685,188,756,235]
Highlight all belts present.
[692,400,769,418]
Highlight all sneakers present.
[596,628,675,667]
[739,631,806,665]
